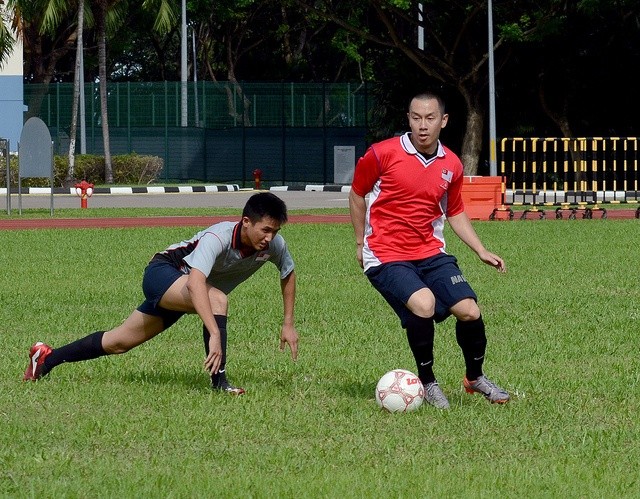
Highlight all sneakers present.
[463,373,510,404]
[22,341,53,382]
[211,380,245,395]
[424,380,450,410]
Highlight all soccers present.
[374,369,426,413]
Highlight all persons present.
[348,92,511,410]
[23,192,299,396]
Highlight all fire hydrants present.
[251,168,263,189]
[75,179,94,209]
